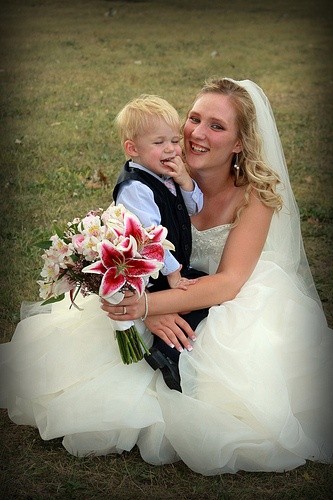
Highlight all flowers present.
[34,200,176,368]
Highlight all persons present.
[110,92,220,392]
[1,75,333,476]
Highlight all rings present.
[122,305,127,315]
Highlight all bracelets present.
[140,290,149,322]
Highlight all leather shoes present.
[143,347,182,395]
